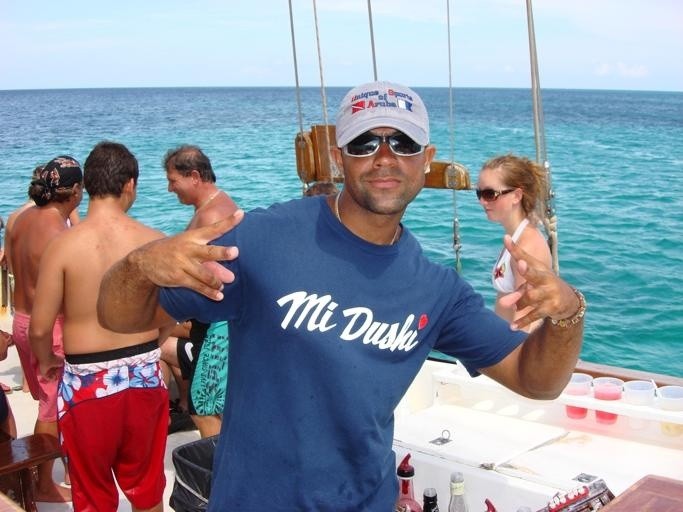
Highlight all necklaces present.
[195,190,222,214]
[334,192,400,247]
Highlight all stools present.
[0,432,64,512]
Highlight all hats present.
[334,80,431,149]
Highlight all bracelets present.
[549,288,587,328]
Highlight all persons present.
[476,155,552,333]
[161,319,211,413]
[0,249,12,393]
[5,165,80,392]
[0,307,17,441]
[96,81,584,512]
[28,141,169,512]
[9,155,82,504]
[162,144,239,439]
[161,360,190,435]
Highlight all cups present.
[593,377,624,426]
[623,380,657,430]
[656,386,683,437]
[564,373,593,420]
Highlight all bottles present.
[447,472,469,512]
[395,454,423,512]
[422,488,439,512]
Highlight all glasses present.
[343,130,425,156]
[475,187,513,202]
